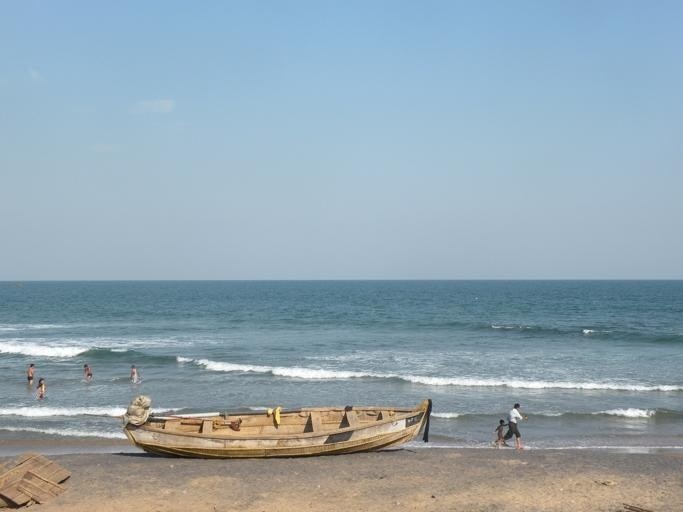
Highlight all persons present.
[84,363,92,380]
[494,418,509,444]
[130,365,138,382]
[36,379,47,400]
[27,364,34,384]
[501,402,527,449]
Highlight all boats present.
[120,395,431,461]
